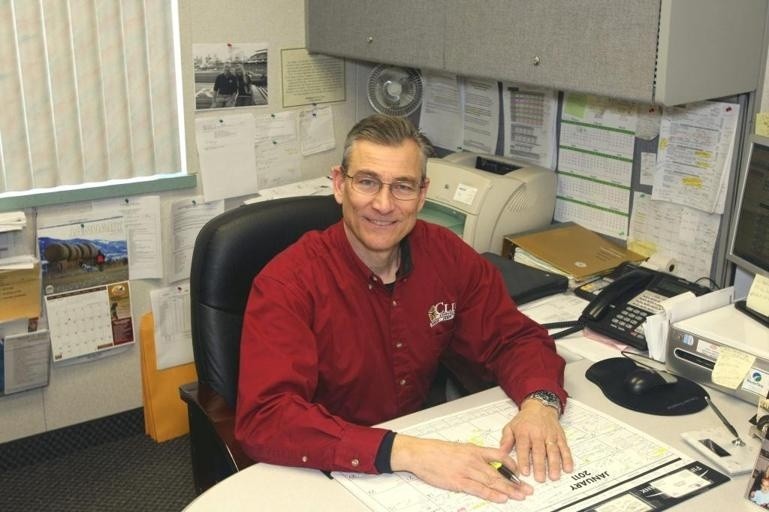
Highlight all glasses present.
[345,170,423,201]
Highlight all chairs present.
[175,192,445,494]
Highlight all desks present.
[178,271,769,512]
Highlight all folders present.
[503,221,647,294]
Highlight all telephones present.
[582,267,711,351]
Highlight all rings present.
[545,438,558,448]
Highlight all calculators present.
[576,261,641,301]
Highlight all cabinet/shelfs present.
[305,1,768,107]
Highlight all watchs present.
[519,390,562,419]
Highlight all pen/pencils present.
[468,436,519,485]
[705,395,739,440]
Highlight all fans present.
[364,65,424,118]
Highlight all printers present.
[416,152,557,256]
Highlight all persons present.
[210,63,237,108]
[749,478,769,507]
[77,262,98,273]
[233,62,253,106]
[95,248,106,272]
[233,114,572,504]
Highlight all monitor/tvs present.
[725,135,769,327]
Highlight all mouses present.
[623,368,657,394]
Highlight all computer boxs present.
[666,300,769,411]
